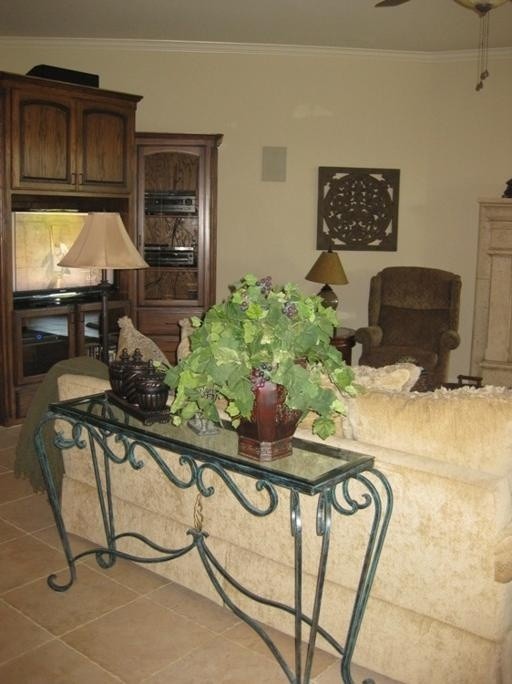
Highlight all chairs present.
[355,264,465,388]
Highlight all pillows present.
[108,313,170,373]
[373,302,450,351]
[347,384,511,471]
[175,316,195,363]
[345,357,428,394]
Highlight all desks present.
[322,324,356,369]
[28,389,395,684]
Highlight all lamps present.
[457,0,509,90]
[301,245,351,313]
[55,211,151,368]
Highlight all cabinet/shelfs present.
[469,195,511,387]
[128,128,226,365]
[0,291,139,429]
[0,69,146,202]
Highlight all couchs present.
[50,359,511,684]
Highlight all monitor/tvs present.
[11,207,121,310]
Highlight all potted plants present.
[157,269,366,464]
